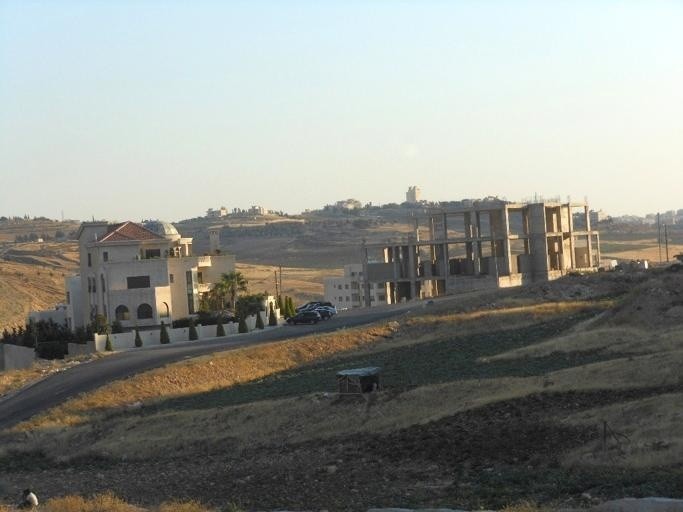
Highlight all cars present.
[285,300,336,324]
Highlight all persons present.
[17,487,38,509]
